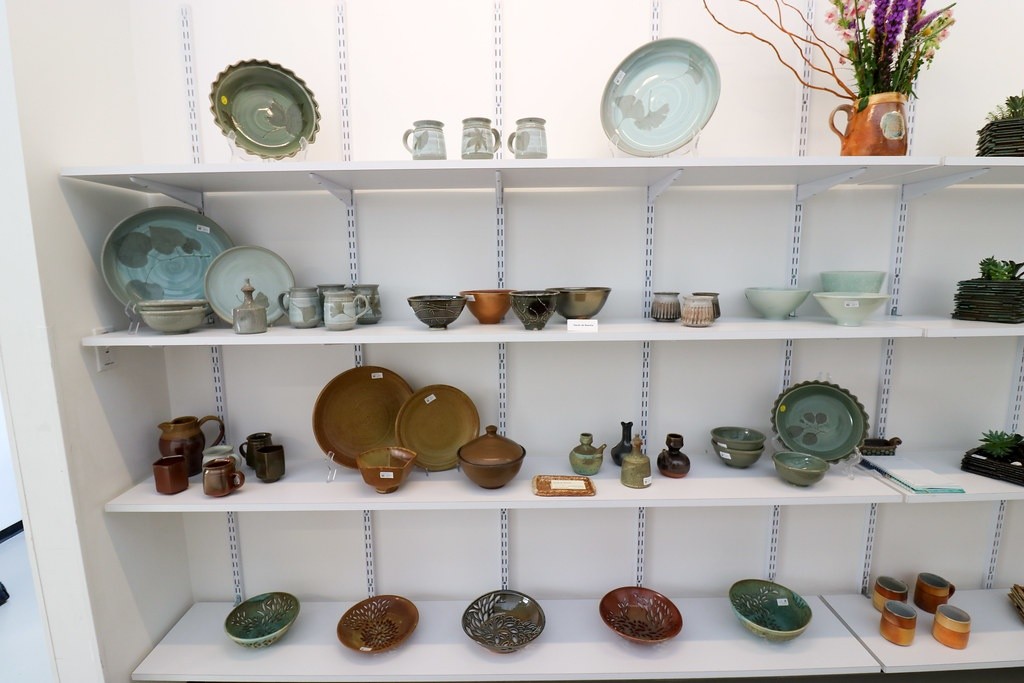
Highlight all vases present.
[826,93,915,156]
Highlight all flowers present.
[702,0,961,115]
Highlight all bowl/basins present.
[744,270,889,325]
[335,595,419,654]
[709,426,830,487]
[457,425,526,489]
[223,591,301,649]
[137,300,206,335]
[599,586,683,646]
[728,579,812,641]
[462,590,546,653]
[355,446,417,493]
[407,287,611,330]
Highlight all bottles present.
[569,421,691,489]
[233,278,267,335]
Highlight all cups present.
[873,575,908,612]
[276,283,381,330]
[402,117,547,159]
[914,572,955,613]
[151,432,285,499]
[880,599,917,646]
[650,291,721,327]
[932,604,971,650]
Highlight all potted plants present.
[974,88,1024,157]
[961,430,1024,488]
[953,255,1024,325]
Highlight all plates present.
[312,365,413,469]
[770,380,870,465]
[394,384,480,471]
[100,205,234,310]
[203,245,295,325]
[209,58,321,160]
[599,36,721,156]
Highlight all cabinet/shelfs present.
[55,149,1024,682]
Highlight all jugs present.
[156,415,224,475]
